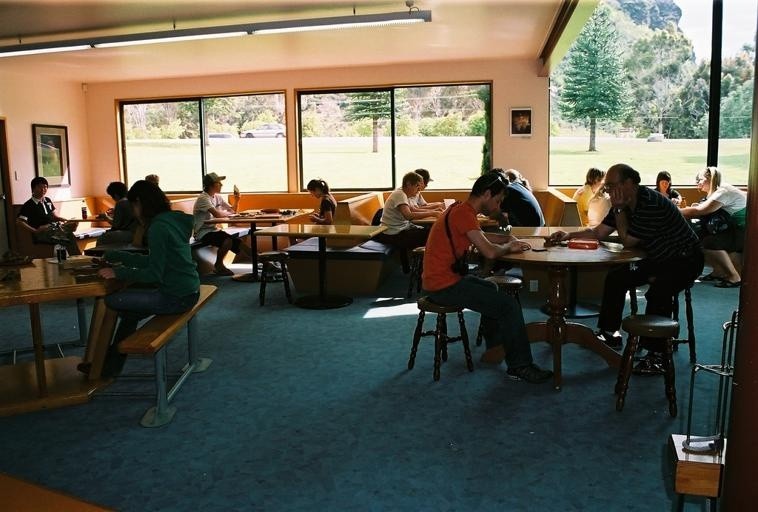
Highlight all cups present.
[55,248,67,263]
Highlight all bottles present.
[680,197,687,208]
[81,200,87,219]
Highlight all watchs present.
[612,207,627,216]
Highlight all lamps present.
[0,11,431,57]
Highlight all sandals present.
[698,273,742,288]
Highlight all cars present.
[240,123,286,138]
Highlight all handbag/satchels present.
[698,197,731,236]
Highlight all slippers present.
[263,264,283,273]
[213,267,234,275]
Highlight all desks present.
[0,256,125,419]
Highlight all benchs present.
[117,284,219,427]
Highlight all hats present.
[203,172,226,183]
[415,169,434,181]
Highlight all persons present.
[487,169,548,276]
[570,166,606,226]
[504,167,532,190]
[289,178,337,247]
[420,168,554,385]
[653,172,684,204]
[192,171,283,277]
[378,172,432,248]
[544,161,705,379]
[75,178,201,378]
[585,181,612,227]
[411,168,446,233]
[676,167,747,288]
[14,176,81,256]
[96,180,141,248]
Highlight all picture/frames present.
[510,107,534,138]
[32,123,71,189]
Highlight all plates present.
[227,208,298,220]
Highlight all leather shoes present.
[77,364,113,377]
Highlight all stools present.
[408,281,696,418]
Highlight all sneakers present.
[594,329,622,351]
[632,352,667,374]
[506,364,553,384]
